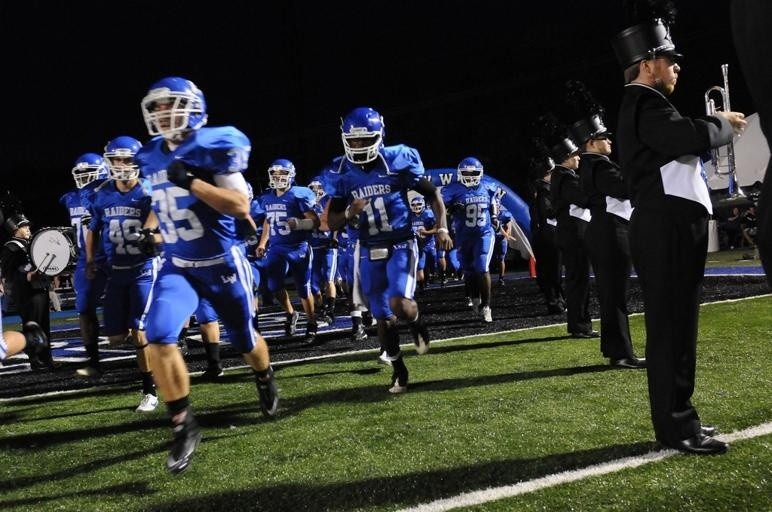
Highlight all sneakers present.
[351,326,368,344]
[425,272,464,290]
[22,318,63,373]
[377,350,391,366]
[135,393,160,413]
[303,322,318,344]
[284,310,300,337]
[139,383,156,393]
[255,376,280,418]
[478,304,493,323]
[497,279,505,286]
[166,413,203,473]
[76,362,104,377]
[464,296,481,313]
[412,326,430,355]
[388,369,410,394]
[201,370,225,378]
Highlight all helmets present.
[456,156,484,188]
[338,106,387,166]
[103,135,143,182]
[140,75,209,140]
[307,175,326,202]
[267,159,297,190]
[406,189,426,214]
[71,153,108,189]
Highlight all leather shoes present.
[571,330,600,339]
[668,431,730,457]
[701,424,716,437]
[608,354,647,370]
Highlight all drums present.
[27,226,80,277]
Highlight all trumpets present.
[704,64,753,210]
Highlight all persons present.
[574,112,650,369]
[616,15,747,457]
[741,204,759,249]
[533,153,566,318]
[726,207,744,250]
[243,158,376,342]
[63,136,225,413]
[131,76,282,475]
[549,136,601,339]
[3,214,55,376]
[317,108,452,397]
[440,156,512,321]
[409,196,464,291]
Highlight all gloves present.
[136,227,157,249]
[165,157,201,192]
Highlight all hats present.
[568,112,614,148]
[534,156,556,176]
[608,16,685,72]
[549,137,580,165]
[4,212,32,234]
